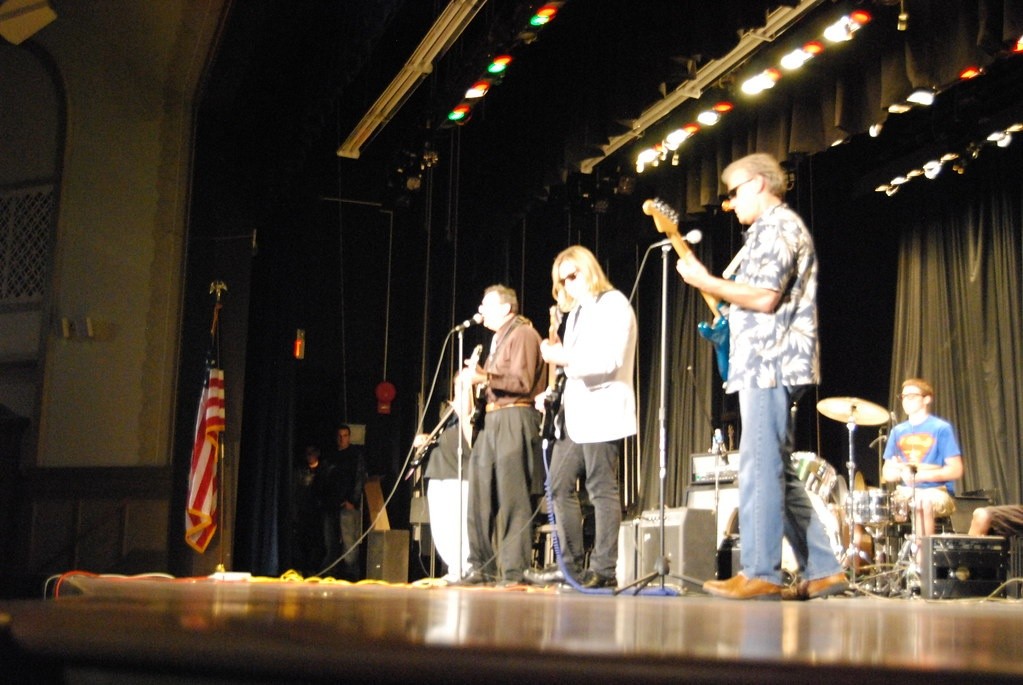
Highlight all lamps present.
[52,315,95,339]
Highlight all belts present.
[486,397,532,412]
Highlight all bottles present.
[712,429,722,454]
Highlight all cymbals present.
[816,396,890,427]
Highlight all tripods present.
[610,247,704,596]
[835,406,923,600]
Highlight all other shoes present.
[497,577,522,590]
[450,571,494,587]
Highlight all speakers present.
[615,452,742,589]
[366,529,411,584]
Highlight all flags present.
[184,303,227,552]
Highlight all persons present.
[447,283,547,587]
[676,152,849,600]
[424,366,472,584]
[523,246,637,589]
[882,377,963,566]
[296,423,365,581]
[968,503,1023,537]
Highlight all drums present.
[790,450,838,506]
[842,489,892,526]
[890,498,909,523]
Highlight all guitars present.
[538,303,563,441]
[642,196,729,383]
[470,343,485,449]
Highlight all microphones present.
[650,229,703,248]
[452,313,483,333]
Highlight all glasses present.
[558,268,580,286]
[898,393,923,399]
[727,177,755,201]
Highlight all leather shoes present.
[703,573,782,602]
[524,564,582,586]
[782,570,849,598]
[580,572,618,589]
